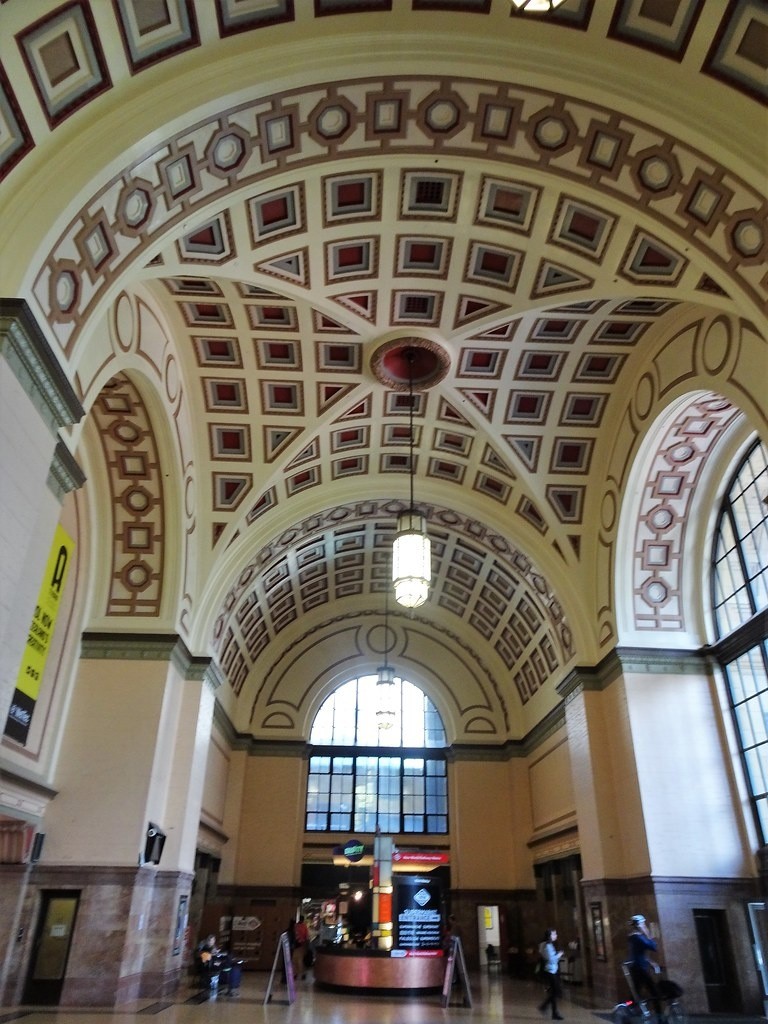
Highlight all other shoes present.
[551,1015,565,1020]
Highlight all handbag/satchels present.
[534,960,543,976]
[303,948,314,967]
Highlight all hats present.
[629,915,647,924]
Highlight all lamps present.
[390,359,433,608]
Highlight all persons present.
[203,936,245,966]
[628,915,663,1014]
[538,928,566,1020]
[281,916,309,984]
[448,915,463,937]
